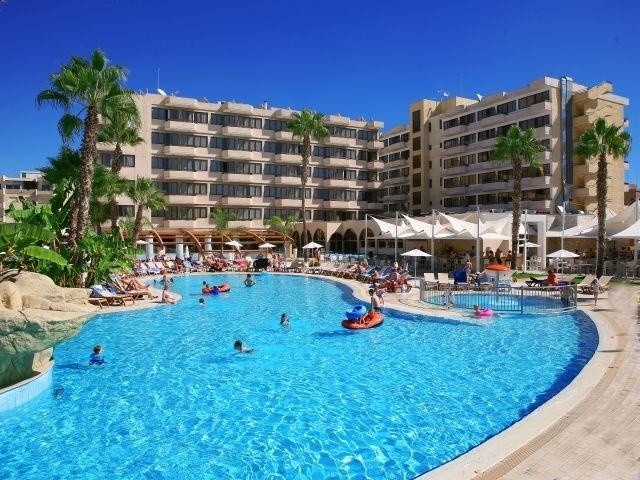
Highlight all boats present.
[342,312,384,329]
[202,284,229,294]
[346,305,366,319]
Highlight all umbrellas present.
[400,248,433,277]
[258,242,277,258]
[302,241,323,258]
[518,242,541,249]
[136,238,149,250]
[224,240,244,260]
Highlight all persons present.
[122,250,411,326]
[89,346,106,366]
[440,245,639,315]
[234,341,253,354]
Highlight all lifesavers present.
[345,305,367,319]
[474,309,491,316]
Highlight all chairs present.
[133,261,208,276]
[526,254,640,281]
[526,275,615,294]
[423,269,471,291]
[284,253,412,293]
[88,277,149,308]
